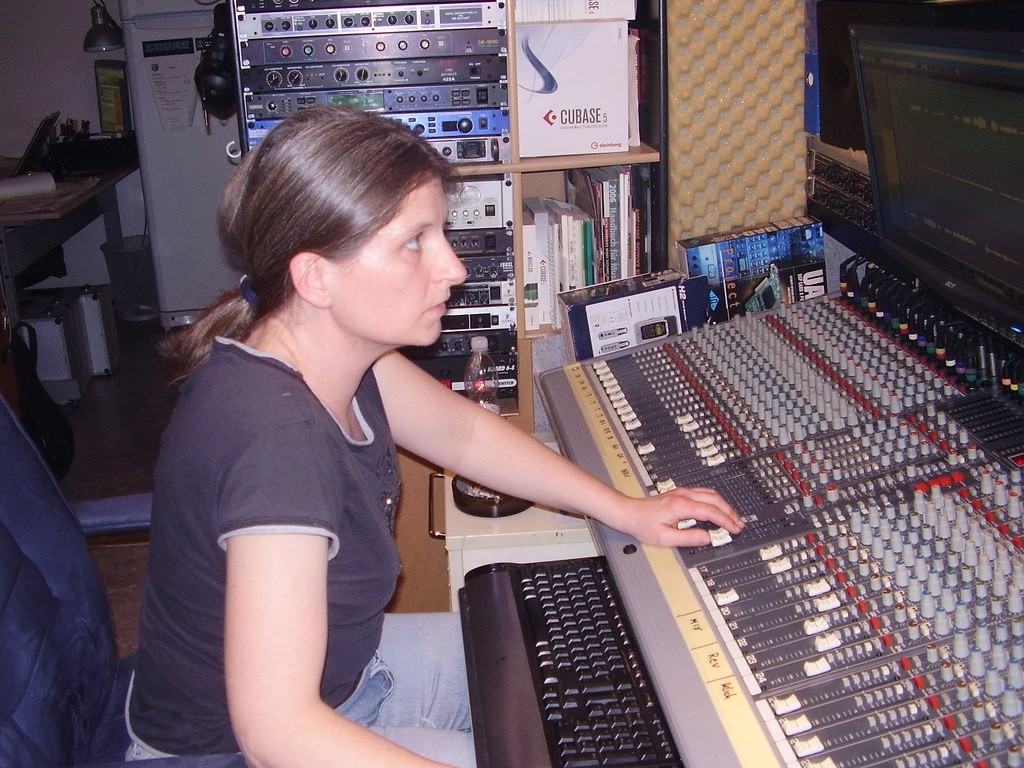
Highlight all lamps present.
[84,5,124,54]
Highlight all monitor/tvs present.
[11,111,62,179]
[94,60,133,134]
[848,22,1024,307]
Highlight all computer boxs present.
[18,284,124,407]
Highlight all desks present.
[0,154,142,331]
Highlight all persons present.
[127,104,746,768]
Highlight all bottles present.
[463,334,501,413]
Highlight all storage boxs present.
[516,17,630,160]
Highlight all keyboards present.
[457,553,684,768]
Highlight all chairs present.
[0,397,134,768]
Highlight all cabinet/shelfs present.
[444,2,662,437]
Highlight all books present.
[522,165,653,330]
[628,27,641,148]
